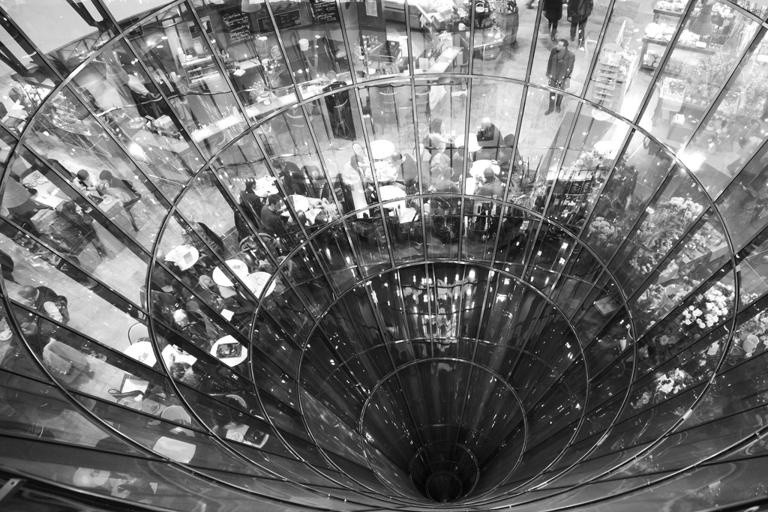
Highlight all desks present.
[119,173,338,464]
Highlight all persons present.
[0,0,604,495]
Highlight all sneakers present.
[545,108,554,115]
[556,105,560,112]
